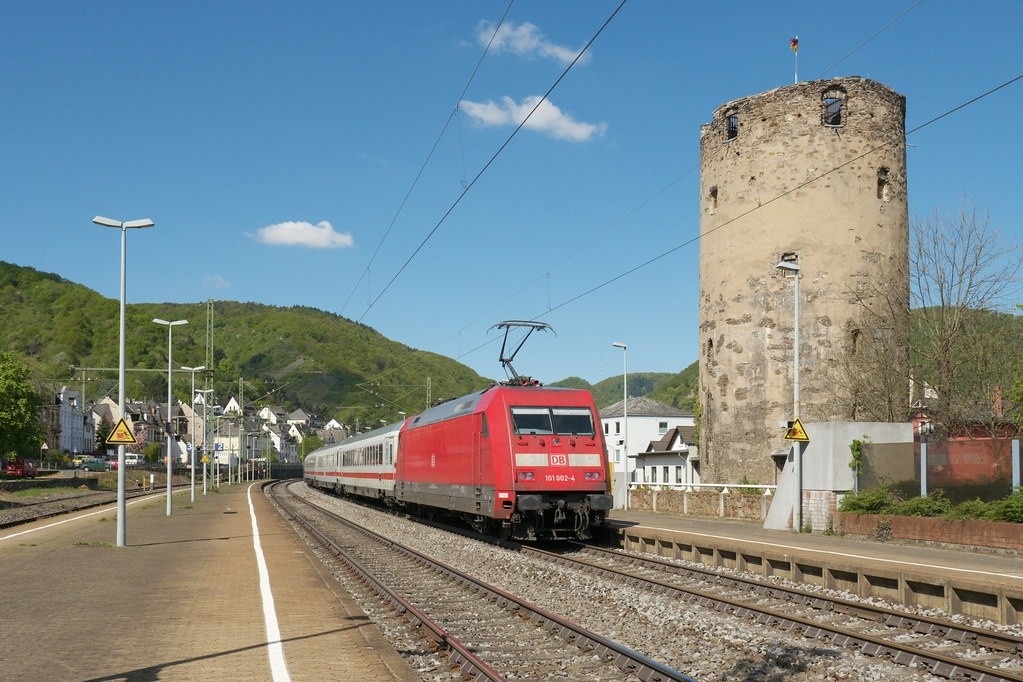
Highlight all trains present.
[303,318,615,544]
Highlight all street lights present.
[613,342,627,510]
[366,427,372,432]
[152,318,190,517]
[228,423,234,486]
[214,414,222,488]
[91,214,153,546]
[238,427,245,483]
[380,419,386,426]
[777,261,801,533]
[252,436,258,482]
[180,365,206,500]
[398,411,406,420]
[246,433,252,484]
[206,405,221,486]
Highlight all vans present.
[5,456,38,480]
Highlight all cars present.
[63,452,147,472]
